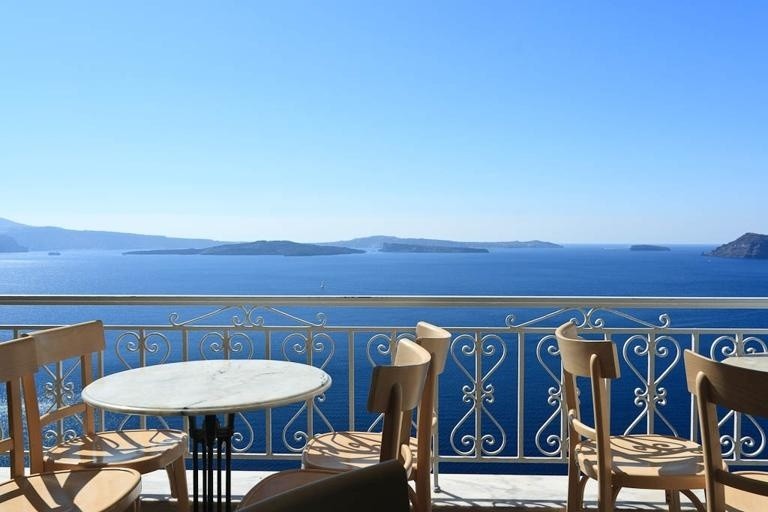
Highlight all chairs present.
[299,320,452,492]
[554,320,729,512]
[233,337,432,512]
[0,336,144,512]
[18,317,192,511]
[684,346,767,512]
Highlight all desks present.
[80,355,333,512]
